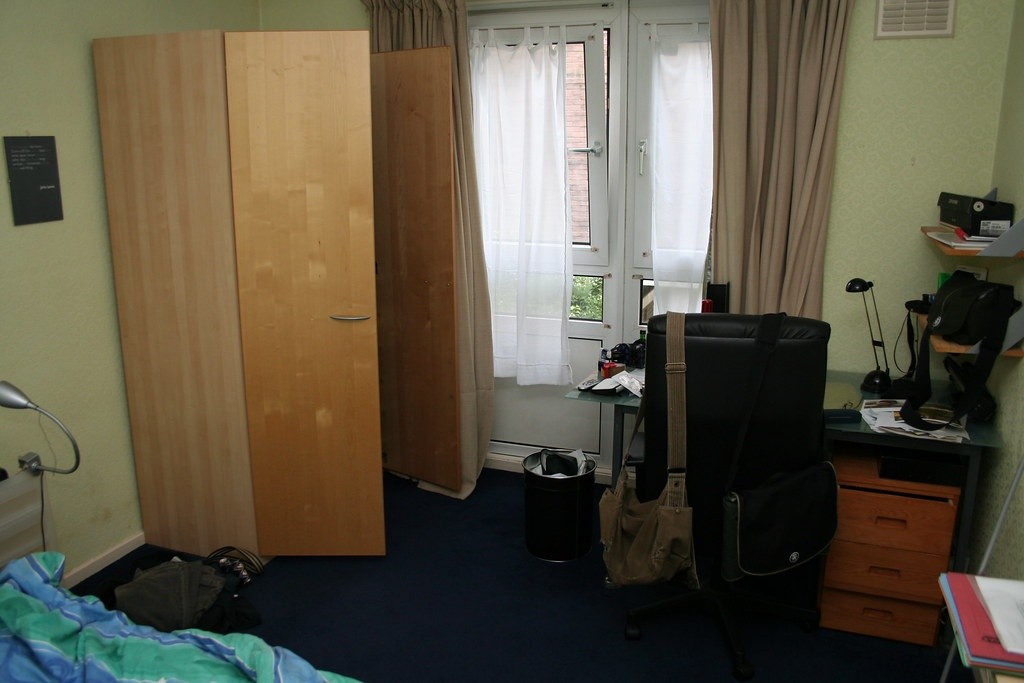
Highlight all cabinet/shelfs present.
[821,474,960,646]
[915,224,1024,357]
[89,28,462,568]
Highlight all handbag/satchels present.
[720,460,839,581]
[926,269,1022,345]
[599,469,698,587]
[115,546,265,633]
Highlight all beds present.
[0,468,362,682]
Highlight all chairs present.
[623,315,838,682]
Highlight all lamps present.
[845,278,892,400]
[0,380,81,476]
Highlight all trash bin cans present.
[521,448,598,563]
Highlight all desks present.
[938,571,1023,683]
[577,346,1007,646]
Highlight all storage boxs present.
[937,191,1015,235]
